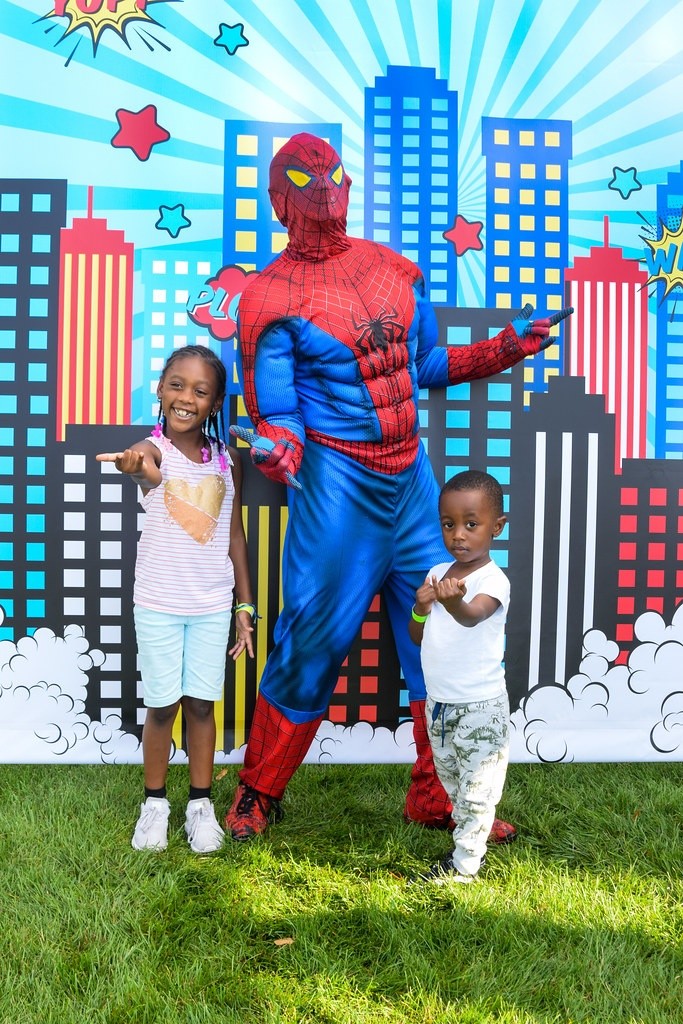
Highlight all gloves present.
[511,303,573,356]
[230,425,304,491]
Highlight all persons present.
[227,132,575,845]
[94,345,255,854]
[408,470,511,886]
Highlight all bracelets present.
[233,603,261,625]
[411,604,428,623]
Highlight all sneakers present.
[402,802,519,845]
[223,779,284,841]
[131,797,170,853]
[445,845,486,871]
[407,859,479,889]
[183,797,226,853]
